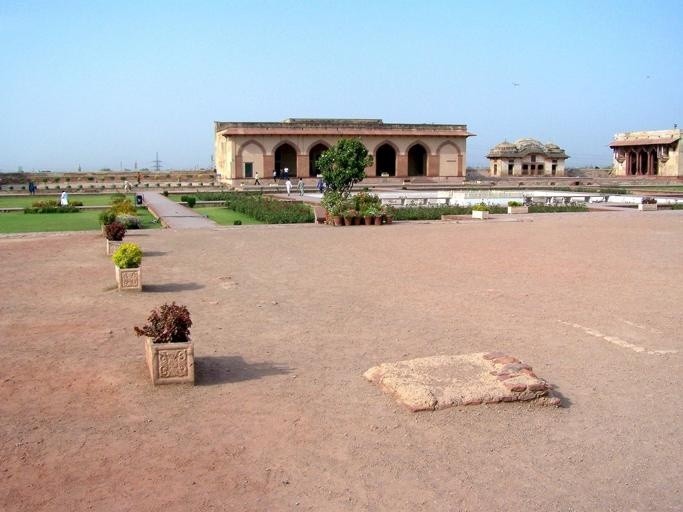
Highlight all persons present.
[254,172,260,185]
[137,172,143,184]
[285,178,293,196]
[60,191,68,207]
[124,179,129,192]
[297,177,305,196]
[279,165,289,180]
[317,179,326,193]
[26,178,35,196]
[272,170,277,182]
[129,181,133,192]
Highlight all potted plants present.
[320,189,393,226]
[113,242,144,291]
[472,205,489,218]
[134,301,195,386]
[508,201,528,214]
[99,211,115,237]
[104,222,126,256]
